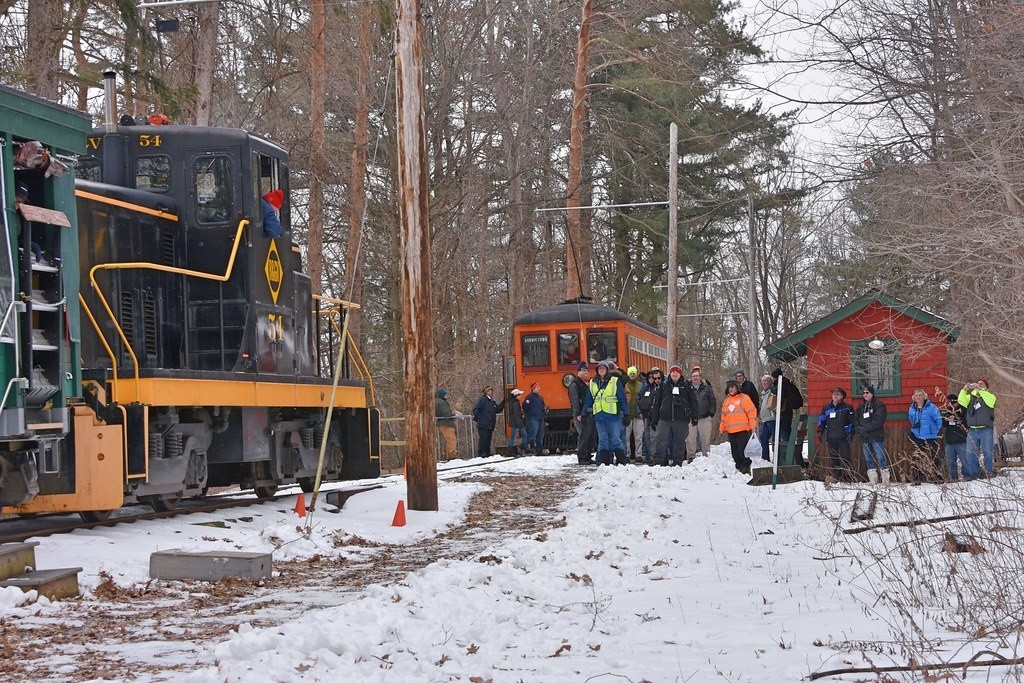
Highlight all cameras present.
[912,422,920,428]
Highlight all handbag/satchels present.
[743,433,762,459]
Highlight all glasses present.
[654,377,661,380]
[863,392,869,394]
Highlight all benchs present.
[772,413,807,469]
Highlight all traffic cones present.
[294,493,307,517]
[392,500,406,526]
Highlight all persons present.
[508,389,535,456]
[719,379,758,475]
[770,368,803,441]
[908,388,944,485]
[262,190,284,238]
[563,342,579,362]
[435,389,457,461]
[942,394,970,483]
[853,386,890,483]
[735,369,759,409]
[473,386,504,458]
[582,361,716,465]
[958,379,996,481]
[568,364,597,464]
[760,375,776,461]
[517,381,548,456]
[817,387,855,483]
[589,335,607,361]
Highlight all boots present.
[881,468,889,483]
[867,468,879,484]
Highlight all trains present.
[501,212,682,457]
[0,67,379,528]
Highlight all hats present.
[669,365,682,375]
[771,369,783,378]
[627,366,637,376]
[577,364,588,371]
[482,386,493,394]
[438,389,447,397]
[947,394,958,404]
[724,380,741,395]
[263,190,284,209]
[831,387,846,399]
[865,386,874,395]
[511,389,523,395]
[529,382,539,390]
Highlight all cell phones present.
[969,383,975,389]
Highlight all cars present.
[993,413,1024,462]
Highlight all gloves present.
[817,431,822,441]
[623,415,631,426]
[845,433,854,444]
[652,421,658,430]
[691,418,698,426]
[581,416,588,426]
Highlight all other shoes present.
[579,453,693,466]
[480,448,549,459]
